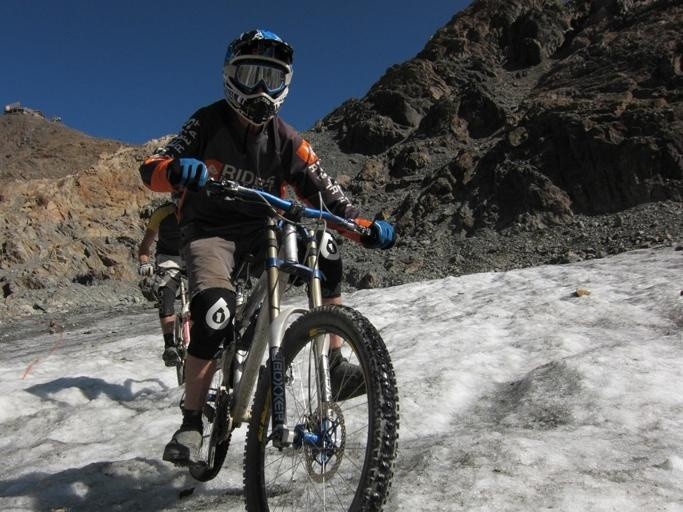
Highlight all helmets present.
[222,31,294,127]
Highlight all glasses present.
[226,55,290,96]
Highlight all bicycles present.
[171,167,407,512]
[138,263,191,385]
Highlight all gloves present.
[137,261,154,277]
[165,157,207,189]
[360,218,396,250]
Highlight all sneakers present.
[331,360,366,401]
[162,344,179,367]
[162,423,203,462]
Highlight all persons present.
[138,26,396,467]
[137,195,187,367]
